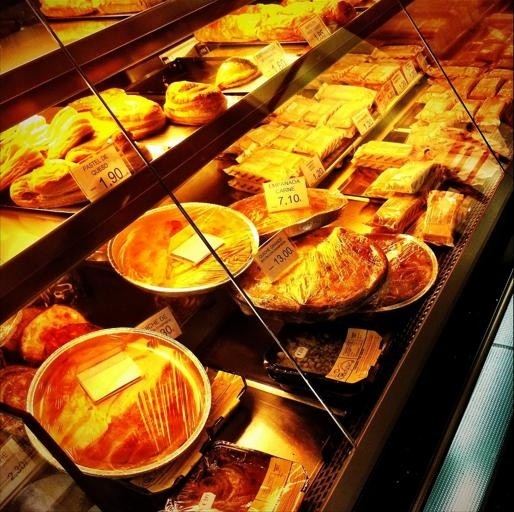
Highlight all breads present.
[0,1,513,512]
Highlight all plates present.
[362,231,438,314]
[106,201,259,298]
[24,327,213,480]
[229,183,348,245]
